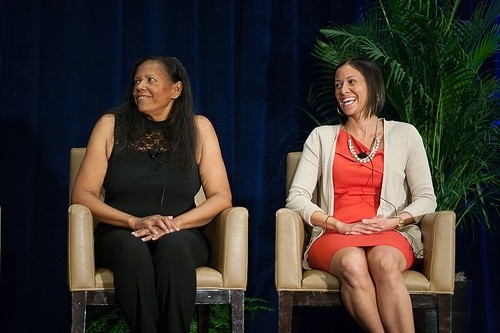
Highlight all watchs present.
[391,215,405,232]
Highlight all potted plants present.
[297,0,500,333]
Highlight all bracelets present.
[325,216,333,232]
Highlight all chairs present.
[68,147,249,333]
[276,152,456,333]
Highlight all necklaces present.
[346,118,383,165]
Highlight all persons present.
[284,56,439,333]
[69,53,234,333]
